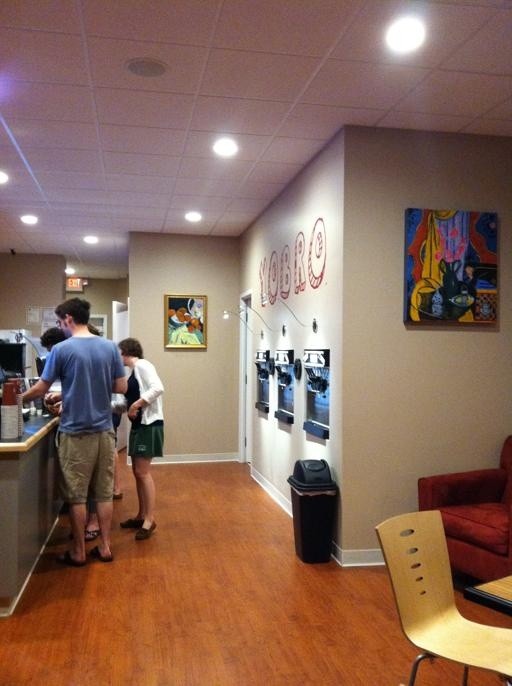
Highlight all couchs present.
[416,434,512,587]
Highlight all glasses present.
[56,317,67,327]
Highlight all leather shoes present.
[134,521,157,540]
[119,518,144,529]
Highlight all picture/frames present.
[403,207,500,331]
[163,294,208,350]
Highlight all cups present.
[0,378,22,438]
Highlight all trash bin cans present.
[287,458,336,564]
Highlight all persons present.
[87,323,124,500]
[21,297,129,566]
[118,337,166,541]
[40,327,101,541]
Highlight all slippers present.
[90,546,114,562]
[85,529,101,540]
[58,550,87,566]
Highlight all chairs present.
[376,511,512,686]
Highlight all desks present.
[0,406,67,595]
